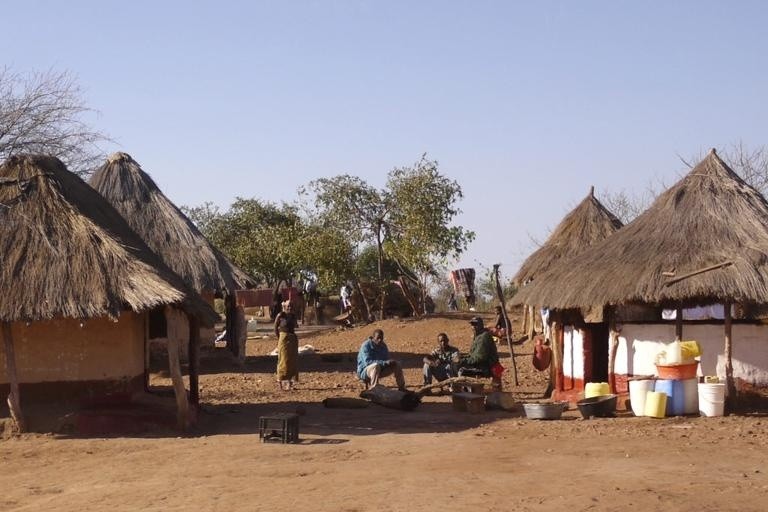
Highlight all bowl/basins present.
[522,401,565,420]
[654,359,702,380]
[576,394,619,420]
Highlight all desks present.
[260,414,300,444]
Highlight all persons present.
[425,295,436,314]
[356,329,411,392]
[272,299,300,390]
[421,333,461,397]
[487,305,513,337]
[445,292,459,311]
[450,317,498,392]
[338,278,355,320]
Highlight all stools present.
[453,392,485,412]
[359,379,369,390]
[498,337,512,345]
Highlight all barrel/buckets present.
[585,382,609,397]
[629,378,725,419]
[247,316,257,331]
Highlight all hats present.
[469,316,483,324]
